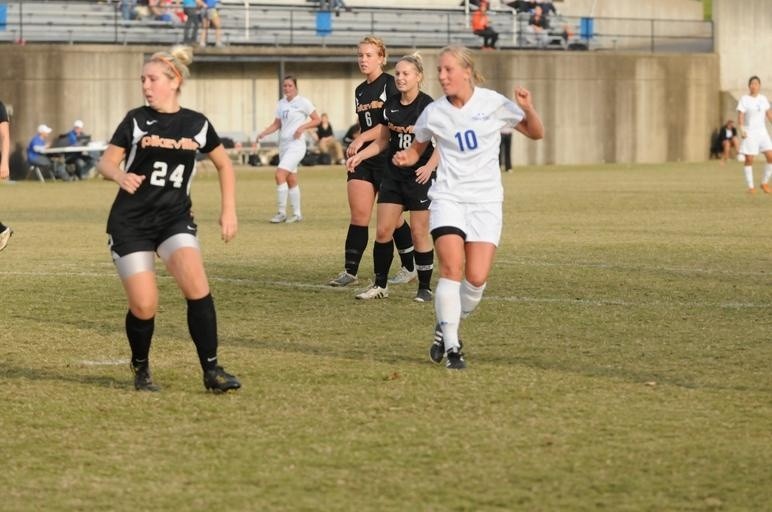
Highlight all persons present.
[23,2,555,182]
[738,76,771,192]
[0,100,15,252]
[391,47,545,371]
[95,44,244,396]
[329,35,415,287]
[254,75,324,224]
[348,53,438,302]
[716,119,740,162]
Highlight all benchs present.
[192,137,350,169]
[0,1,596,52]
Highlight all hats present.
[73,120,84,127]
[38,124,52,134]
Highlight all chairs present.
[24,148,57,182]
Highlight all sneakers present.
[413,288,433,303]
[130,357,160,392]
[387,265,417,284]
[329,271,358,287]
[0,226,14,251]
[204,365,240,394]
[430,322,444,363]
[446,346,467,369]
[355,285,388,299]
[284,215,302,224]
[270,213,287,222]
[750,188,756,194]
[760,184,771,193]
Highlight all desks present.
[36,144,123,181]
